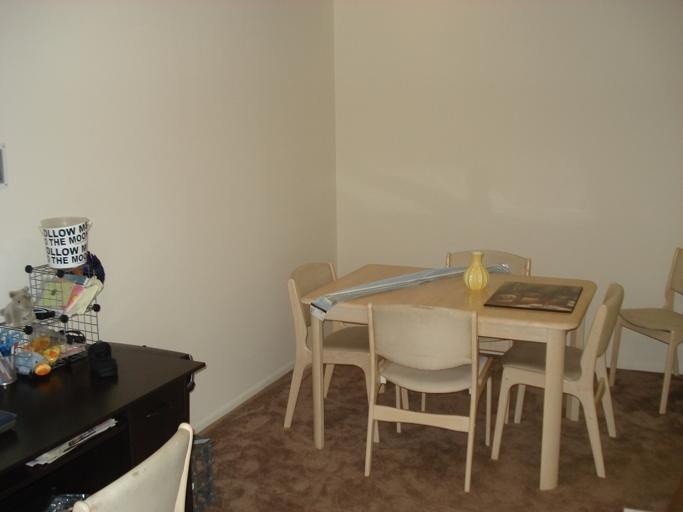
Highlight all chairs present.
[604,247,682,420]
[65,421,195,512]
[491,278,629,482]
[360,298,496,494]
[395,249,533,446]
[278,256,406,442]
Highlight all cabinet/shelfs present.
[0,255,103,375]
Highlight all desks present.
[0,336,206,512]
[299,259,598,493]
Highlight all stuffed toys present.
[0,286,58,378]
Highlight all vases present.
[463,250,487,292]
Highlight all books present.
[483,280,584,313]
[31,272,104,317]
[24,416,118,467]
